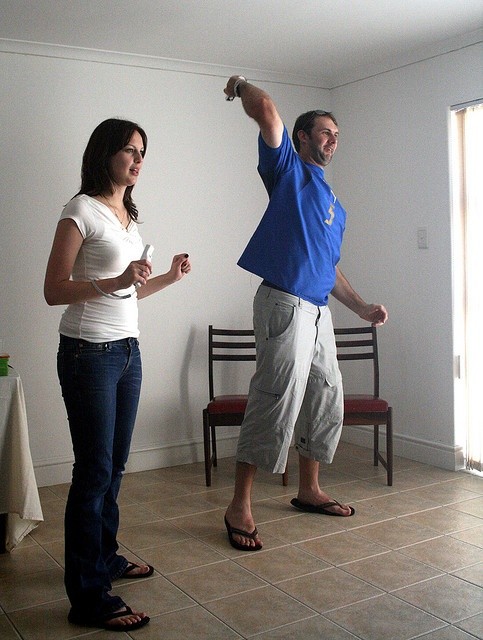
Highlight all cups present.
[0,353,10,376]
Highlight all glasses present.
[303,110,337,128]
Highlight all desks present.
[0,366,45,554]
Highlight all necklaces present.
[107,197,125,225]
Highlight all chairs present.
[201,323,289,488]
[333,320,393,484]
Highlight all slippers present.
[224,513,263,551]
[121,562,154,579]
[101,606,150,632]
[290,494,355,517]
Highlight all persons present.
[43,118,191,632]
[223,75,388,551]
